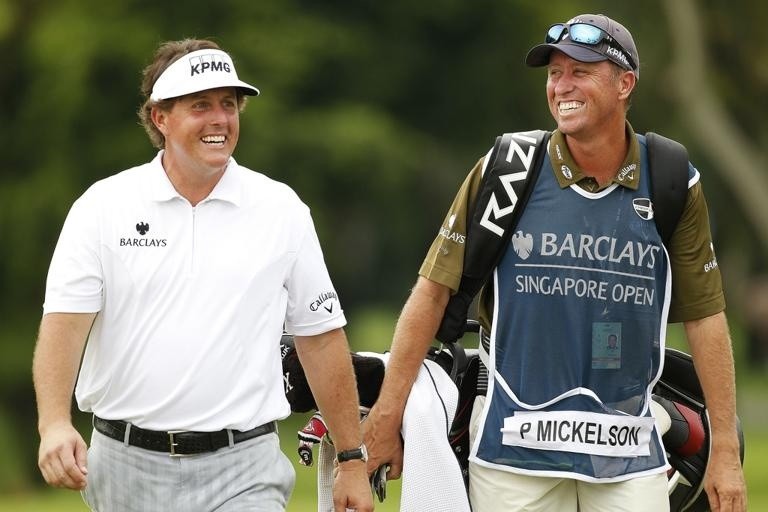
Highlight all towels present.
[318,351,473,512]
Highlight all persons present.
[333,15,747,512]
[33,37,375,512]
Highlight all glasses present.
[545,22,637,71]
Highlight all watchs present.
[335,443,369,463]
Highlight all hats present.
[524,13,640,81]
[151,48,259,102]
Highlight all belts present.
[91,415,276,456]
